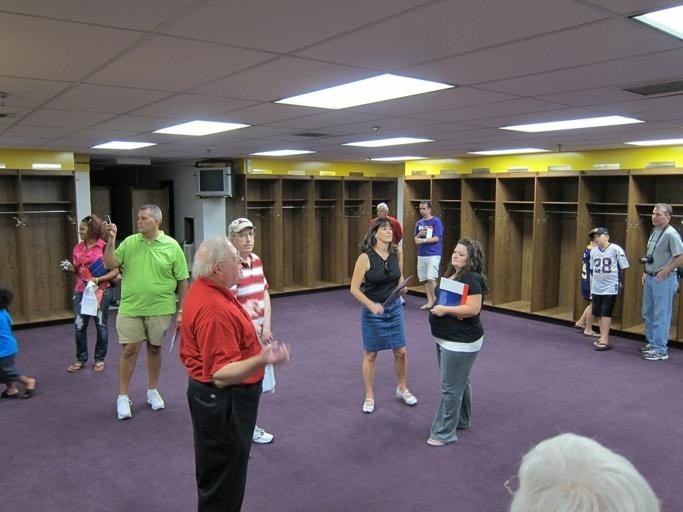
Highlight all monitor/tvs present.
[193,168,233,198]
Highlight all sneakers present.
[642,349,670,361]
[117,394,132,420]
[639,344,653,352]
[146,388,165,411]
[396,385,418,405]
[362,398,375,413]
[251,426,274,444]
[427,437,447,447]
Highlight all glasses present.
[231,233,255,238]
[382,259,390,277]
[502,475,520,495]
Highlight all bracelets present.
[423,239,426,244]
[93,276,98,284]
[177,309,182,313]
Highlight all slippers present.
[0,389,20,398]
[93,361,105,372]
[22,379,39,399]
[67,363,83,372]
[574,322,612,351]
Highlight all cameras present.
[61,262,71,272]
[639,255,654,264]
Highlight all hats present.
[228,218,257,238]
[589,228,609,237]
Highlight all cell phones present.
[105,215,111,224]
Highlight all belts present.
[643,269,675,276]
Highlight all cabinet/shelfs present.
[222,173,399,298]
[401,169,683,346]
[0,167,81,330]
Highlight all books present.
[381,275,413,308]
[436,276,469,322]
[418,224,432,238]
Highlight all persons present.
[499,431,662,511]
[426,238,488,445]
[588,225,630,351]
[350,217,417,413]
[413,201,443,309]
[637,203,682,361]
[369,203,406,304]
[573,239,601,337]
[227,217,276,444]
[102,204,189,421]
[59,215,113,374]
[0,288,37,400]
[176,236,289,510]
